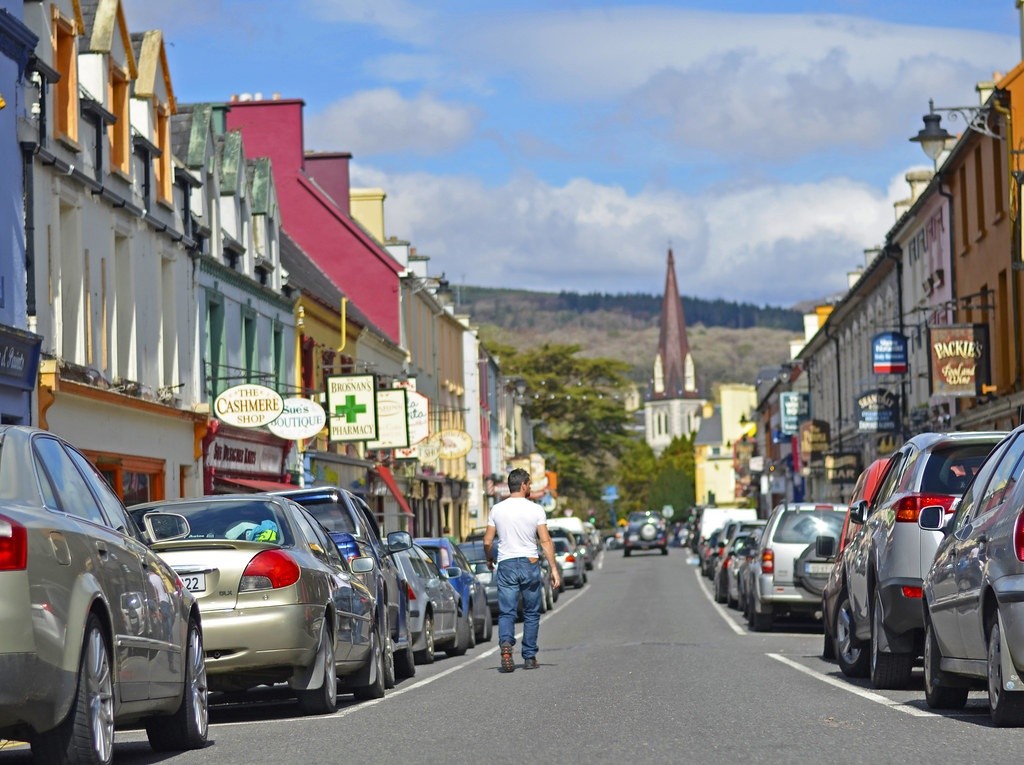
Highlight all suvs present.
[268,485,418,686]
[813,455,978,659]
[831,430,1012,691]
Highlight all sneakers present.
[500,641,515,671]
[525,658,540,669]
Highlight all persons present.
[482,468,560,673]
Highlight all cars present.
[1,424,211,764]
[918,424,1023,725]
[382,504,845,664]
[122,493,389,714]
